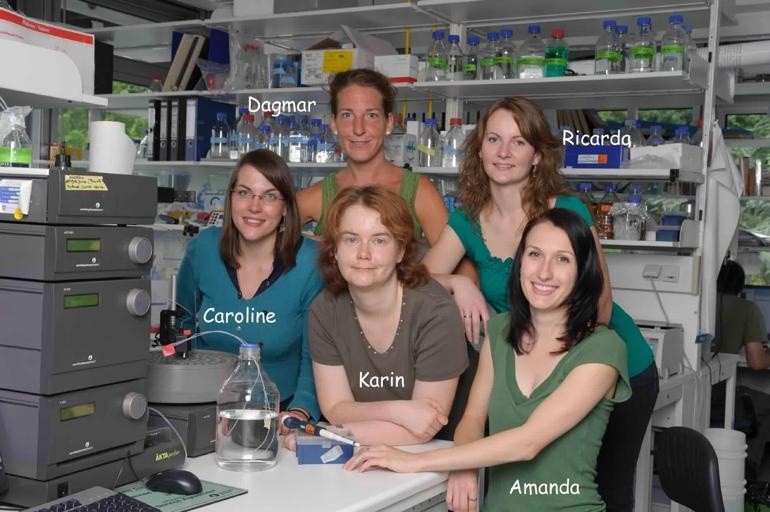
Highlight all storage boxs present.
[300,21,398,87]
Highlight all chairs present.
[655,427,725,512]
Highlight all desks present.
[183,420,484,512]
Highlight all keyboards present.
[20,485,161,512]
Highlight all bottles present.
[133,129,149,177]
[576,180,660,240]
[146,75,165,94]
[214,342,281,474]
[209,107,703,168]
[426,14,697,81]
[0,111,33,167]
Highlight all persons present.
[419,97,659,512]
[712,261,768,481]
[343,208,632,512]
[288,68,478,290]
[284,187,470,451]
[174,150,327,434]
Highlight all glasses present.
[230,190,286,204]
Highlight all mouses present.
[145,469,202,495]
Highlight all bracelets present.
[289,409,309,420]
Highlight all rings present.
[463,314,472,319]
[468,497,478,502]
